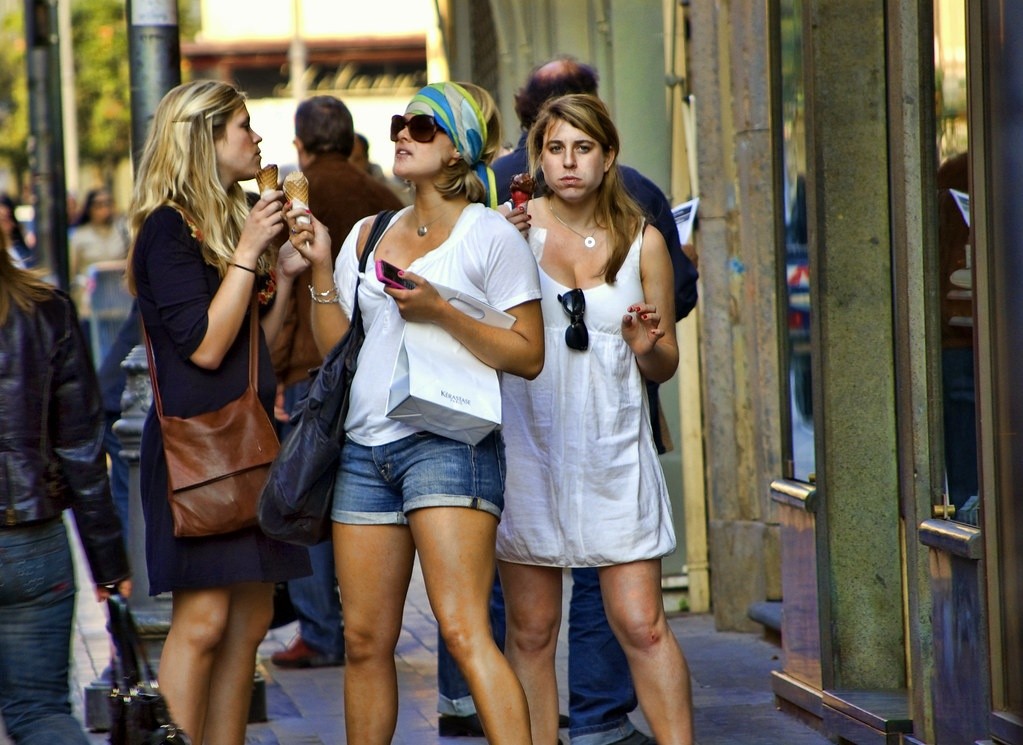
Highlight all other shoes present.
[437,713,484,736]
[271,638,346,667]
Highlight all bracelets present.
[308,285,340,303]
[228,263,257,274]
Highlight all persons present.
[244,94,413,672]
[495,94,694,745]
[938,153,978,519]
[126,80,312,745]
[783,173,814,428]
[0,188,148,745]
[436,56,700,745]
[281,83,545,745]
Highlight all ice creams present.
[255,164,278,193]
[509,172,538,207]
[283,171,309,207]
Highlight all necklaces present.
[414,199,463,236]
[550,197,603,247]
[181,209,277,305]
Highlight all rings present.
[292,225,300,235]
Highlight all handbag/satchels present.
[158,389,282,537]
[386,282,517,447]
[107,595,188,745]
[259,211,405,546]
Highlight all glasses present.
[390,114,447,143]
[557,287,589,351]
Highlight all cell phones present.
[376,259,417,291]
